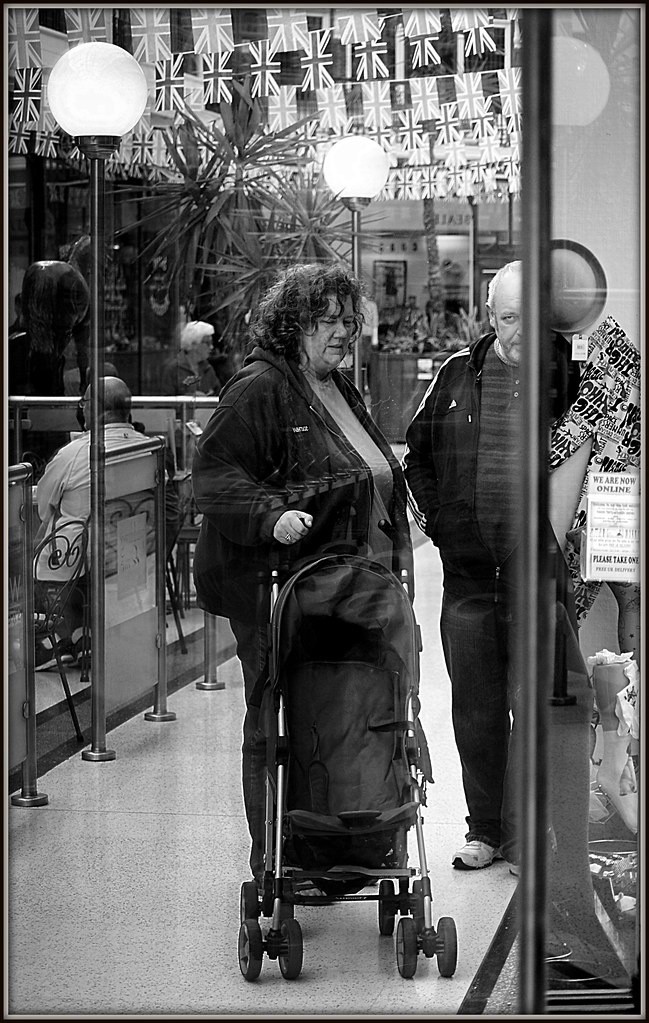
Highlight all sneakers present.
[72,635,92,668]
[35,638,73,671]
[453,840,505,871]
[507,862,520,875]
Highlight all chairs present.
[30,474,192,745]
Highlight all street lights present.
[45,41,147,765]
[320,134,394,397]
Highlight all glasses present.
[79,397,90,408]
[201,340,213,345]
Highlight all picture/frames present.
[373,260,407,311]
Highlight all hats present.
[548,238,608,332]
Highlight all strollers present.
[237,517,459,985]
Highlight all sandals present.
[257,882,330,904]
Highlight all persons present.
[399,259,526,871]
[35,374,154,672]
[183,261,417,907]
[153,320,224,398]
[358,290,380,400]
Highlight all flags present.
[7,8,522,203]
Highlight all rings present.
[285,533,291,541]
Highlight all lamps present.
[46,43,149,158]
[324,135,391,211]
